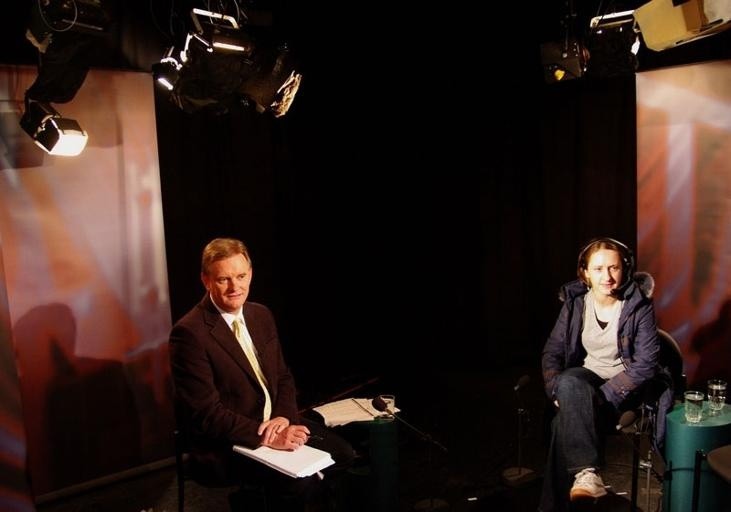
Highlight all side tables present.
[662,401,731,511]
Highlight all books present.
[233,445,335,478]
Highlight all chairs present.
[176,393,270,511]
[548,329,687,512]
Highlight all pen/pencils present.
[307,434,314,436]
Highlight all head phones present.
[580,238,635,276]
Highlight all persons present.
[538,238,675,512]
[168,238,361,512]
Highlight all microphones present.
[371,396,451,511]
[495,374,538,487]
[611,278,630,295]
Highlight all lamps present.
[16,99,88,158]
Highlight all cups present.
[707,378,728,411]
[684,390,706,423]
[377,393,396,419]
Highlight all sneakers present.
[570,469,607,506]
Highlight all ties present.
[233,318,270,390]
